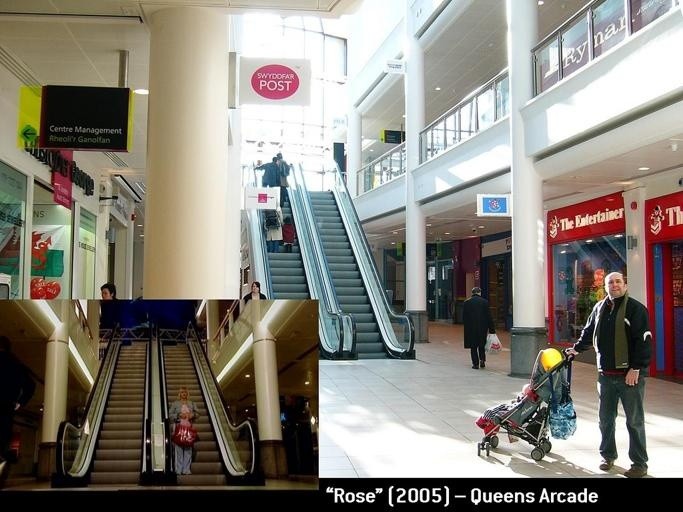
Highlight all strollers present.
[478,349,575,461]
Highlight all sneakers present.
[600,459,615,471]
[624,463,648,478]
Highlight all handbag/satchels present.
[171,423,198,450]
[548,362,577,440]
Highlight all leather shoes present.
[472,359,486,369]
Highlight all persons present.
[281,215,296,253]
[380,166,393,185]
[272,152,290,208]
[165,385,199,477]
[563,269,655,482]
[462,287,495,370]
[242,281,266,304]
[100,283,118,300]
[260,206,285,253]
[253,156,279,188]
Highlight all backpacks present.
[263,210,281,232]
[279,159,291,177]
